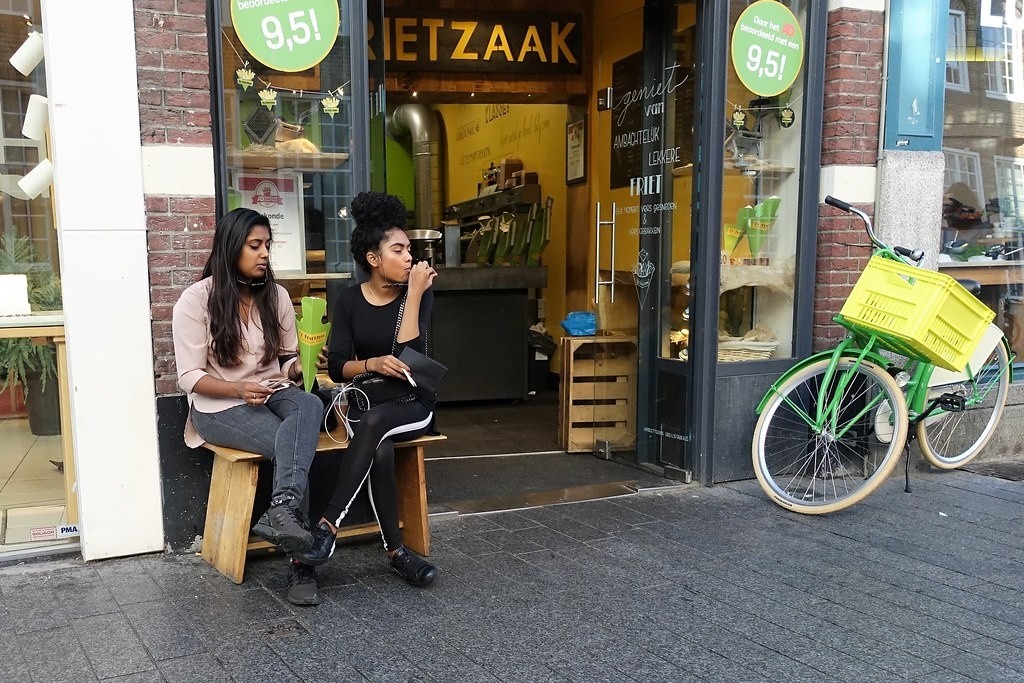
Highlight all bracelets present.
[293,362,299,376]
[365,359,370,373]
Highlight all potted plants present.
[1,222,63,436]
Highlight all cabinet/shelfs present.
[226,148,353,281]
[670,161,795,286]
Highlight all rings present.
[253,400,256,405]
[254,393,256,398]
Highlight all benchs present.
[200,426,447,583]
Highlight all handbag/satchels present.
[351,371,418,412]
[279,352,340,432]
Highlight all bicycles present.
[750,194,1022,515]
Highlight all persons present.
[171,209,325,605]
[296,192,439,587]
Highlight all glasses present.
[233,270,268,288]
[378,254,409,288]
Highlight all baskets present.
[835,249,997,371]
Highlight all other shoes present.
[387,546,437,588]
[297,521,338,565]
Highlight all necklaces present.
[367,280,398,300]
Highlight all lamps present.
[8,30,54,199]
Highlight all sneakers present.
[251,500,314,555]
[285,560,320,605]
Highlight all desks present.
[938,259,1024,284]
[0,309,78,524]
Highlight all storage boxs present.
[838,252,997,373]
[559,337,637,453]
[499,157,538,188]
[982,300,1023,365]
